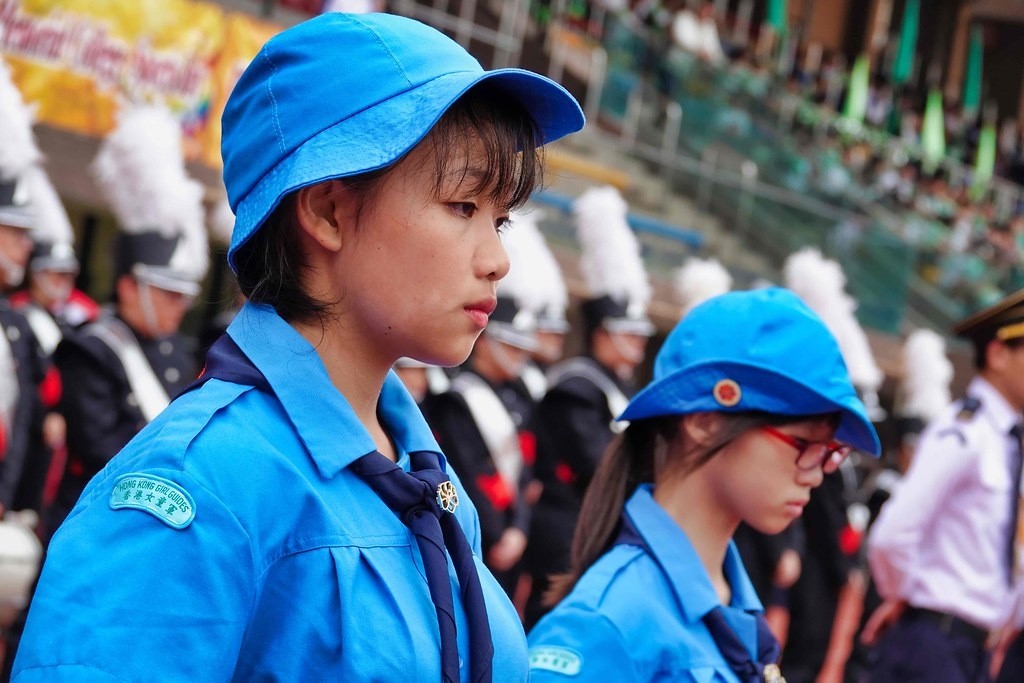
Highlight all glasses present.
[762,425,853,474]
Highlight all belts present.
[904,604,991,640]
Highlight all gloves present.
[1,521,43,609]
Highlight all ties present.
[1008,424,1024,589]
[170,332,494,683]
[617,510,780,683]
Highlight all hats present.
[530,229,568,334]
[30,173,81,272]
[91,104,212,295]
[0,60,36,229]
[614,287,883,457]
[952,288,1024,343]
[571,183,655,335]
[221,12,586,278]
[893,329,954,443]
[486,205,537,351]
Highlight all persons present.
[528,285,882,682]
[1,1,1024,683]
[10,9,586,683]
